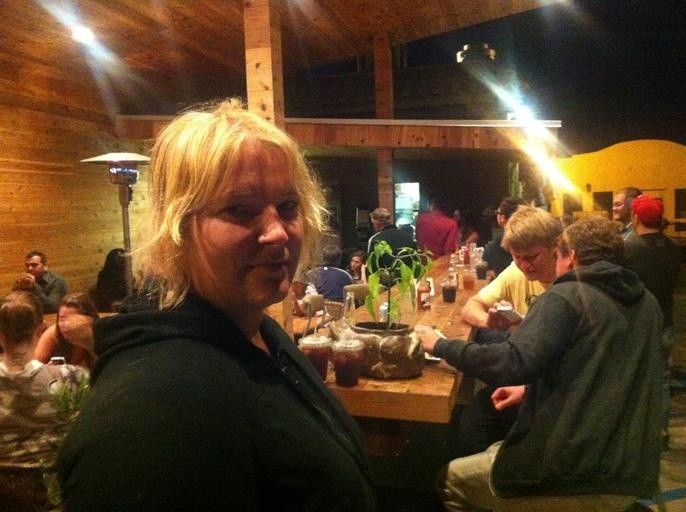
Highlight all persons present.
[300,187,684,512]
[57,96,375,511]
[1,246,128,511]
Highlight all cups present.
[297,334,366,388]
[439,270,477,302]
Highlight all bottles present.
[415,273,431,312]
[448,242,471,290]
[341,291,358,337]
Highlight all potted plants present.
[346,239,436,379]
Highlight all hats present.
[632,195,664,222]
